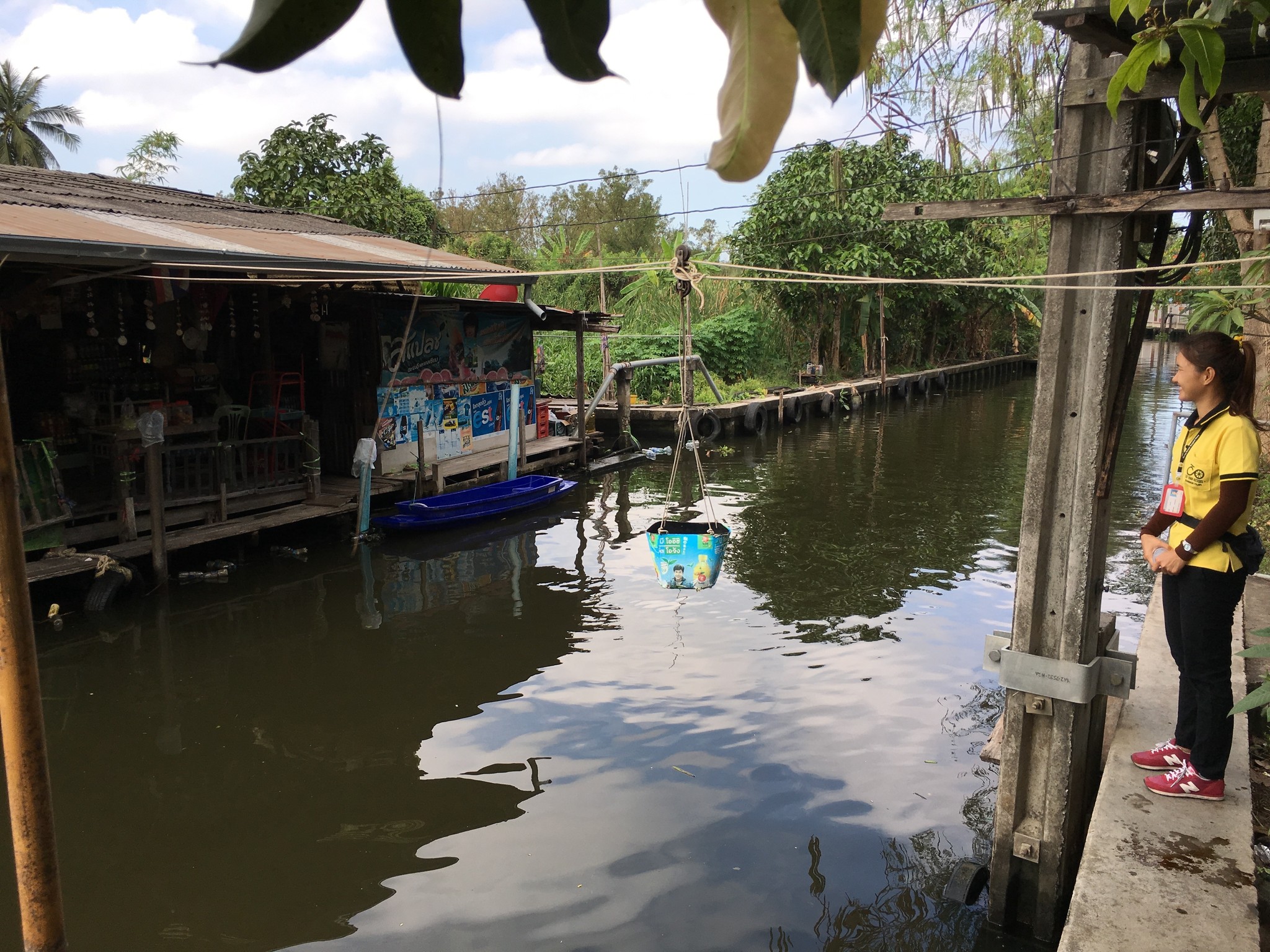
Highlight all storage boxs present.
[536,403,549,439]
[164,364,195,393]
[188,361,219,389]
[549,418,571,436]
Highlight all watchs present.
[1182,540,1200,555]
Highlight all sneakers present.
[1131,738,1190,771]
[1143,761,1225,801]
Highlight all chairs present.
[211,403,252,490]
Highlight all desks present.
[85,416,219,507]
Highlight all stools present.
[171,447,213,497]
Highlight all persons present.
[460,314,524,378]
[1171,490,1183,499]
[1129,331,1266,802]
[666,564,695,589]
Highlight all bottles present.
[175,400,193,425]
[148,401,168,427]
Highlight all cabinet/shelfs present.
[36,335,171,476]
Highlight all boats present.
[368,473,578,541]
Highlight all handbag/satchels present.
[1228,525,1266,575]
